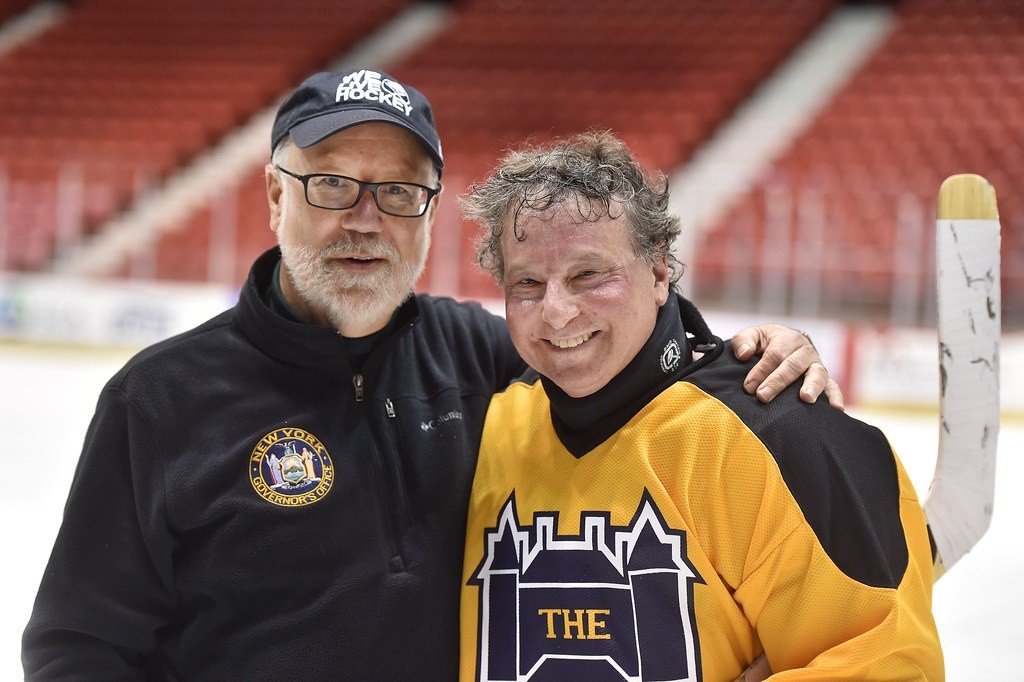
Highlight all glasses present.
[275,162,442,218]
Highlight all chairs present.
[0,0,93,272]
[691,0,1024,333]
[94,0,690,304]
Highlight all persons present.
[19,69,847,681]
[458,131,947,681]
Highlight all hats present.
[269,68,444,180]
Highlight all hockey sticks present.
[732,173,1003,682]
